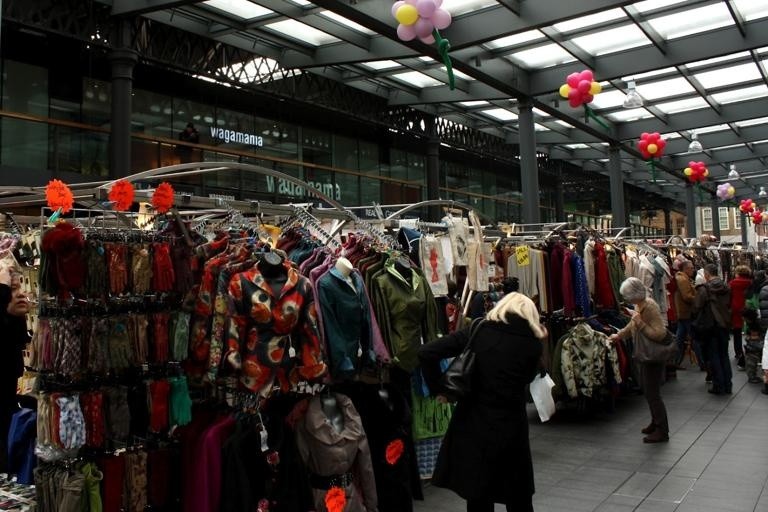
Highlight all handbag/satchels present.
[633,328,678,365]
[443,348,477,400]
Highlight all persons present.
[373,257,438,365]
[670,234,768,395]
[295,393,378,512]
[360,391,425,512]
[416,291,549,512]
[608,277,669,443]
[0,263,33,473]
[177,122,204,165]
[319,255,374,369]
[224,253,326,393]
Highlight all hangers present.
[218,379,280,455]
[546,221,671,269]
[354,216,416,282]
[216,206,290,282]
[278,375,343,420]
[537,308,618,339]
[285,205,357,274]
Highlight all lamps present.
[623,81,643,108]
[728,165,740,179]
[759,187,767,196]
[688,131,703,153]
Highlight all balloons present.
[684,161,710,183]
[739,199,768,224]
[716,183,735,201]
[639,132,665,159]
[559,70,601,108]
[392,0,454,90]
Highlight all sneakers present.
[642,355,767,444]
